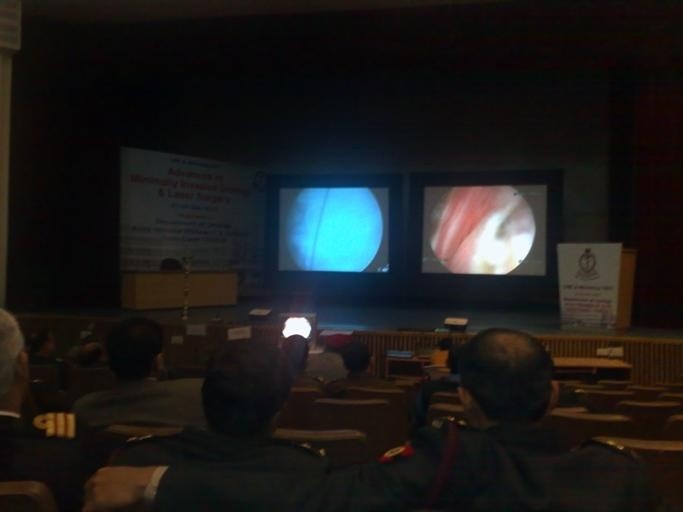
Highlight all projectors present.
[250,308,271,318]
[444,316,468,329]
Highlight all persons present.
[1,308,657,511]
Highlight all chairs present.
[0,378,683,512]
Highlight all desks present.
[386,358,431,381]
[553,357,634,382]
